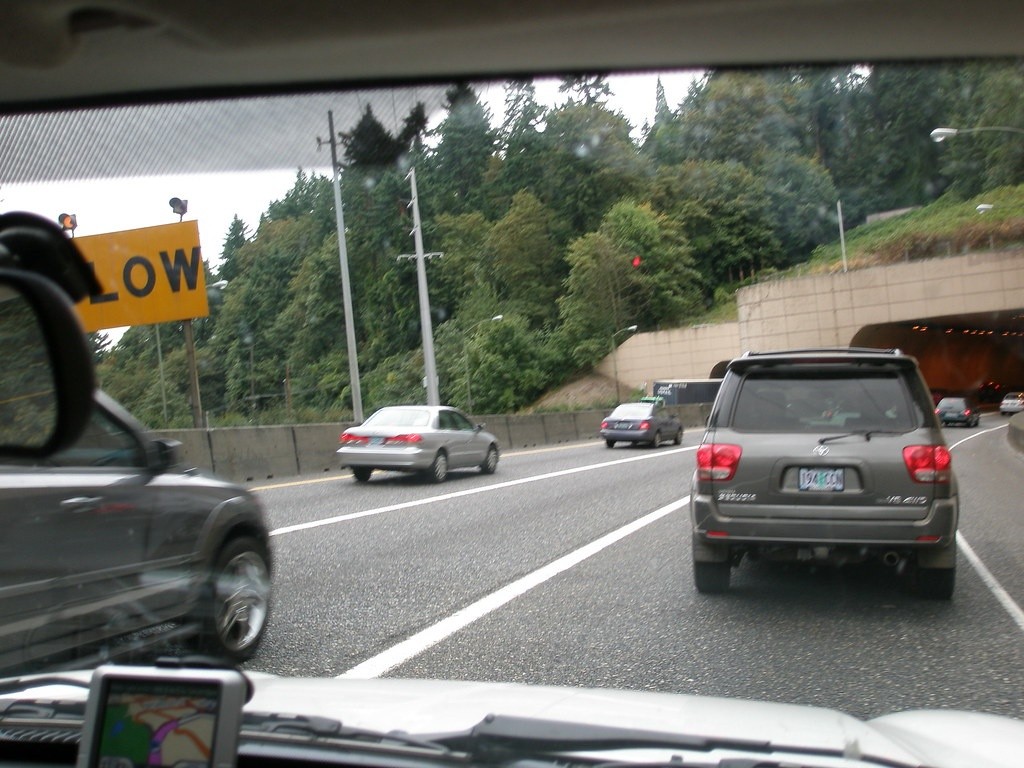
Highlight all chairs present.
[845,404,899,432]
[742,389,802,429]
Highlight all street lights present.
[612,324,638,404]
[463,314,504,415]
[396,165,446,405]
[155,278,229,426]
[316,132,365,427]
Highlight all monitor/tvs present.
[76,665,246,768]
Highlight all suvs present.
[690,354,961,600]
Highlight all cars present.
[600,402,685,449]
[338,406,499,483]
[0,388,275,680]
[999,393,1024,415]
[933,397,981,428]
[978,382,1009,402]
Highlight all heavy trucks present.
[639,377,723,406]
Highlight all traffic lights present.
[168,198,188,216]
[58,213,77,230]
